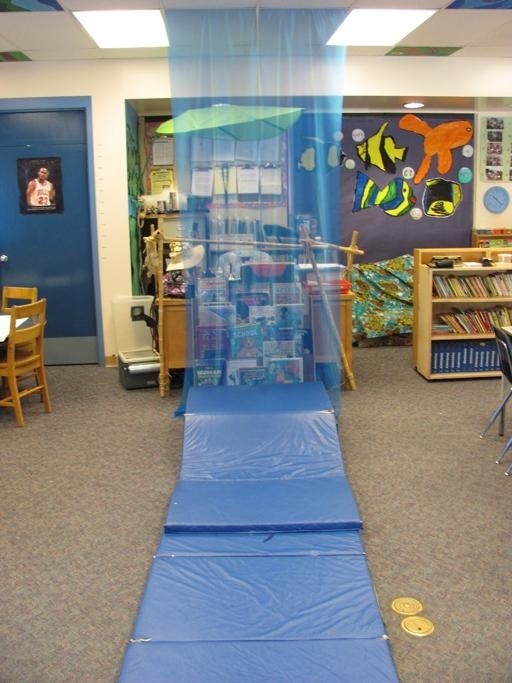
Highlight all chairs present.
[2,287,43,402]
[0,298,51,427]
[480,324,512,464]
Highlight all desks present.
[499,326,512,436]
[1,314,47,348]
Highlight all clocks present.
[484,186,508,212]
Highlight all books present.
[432,274,512,335]
[194,277,304,386]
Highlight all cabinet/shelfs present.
[472,232,512,248]
[155,290,355,396]
[413,248,512,380]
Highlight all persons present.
[26,168,56,208]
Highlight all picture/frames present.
[17,157,64,214]
